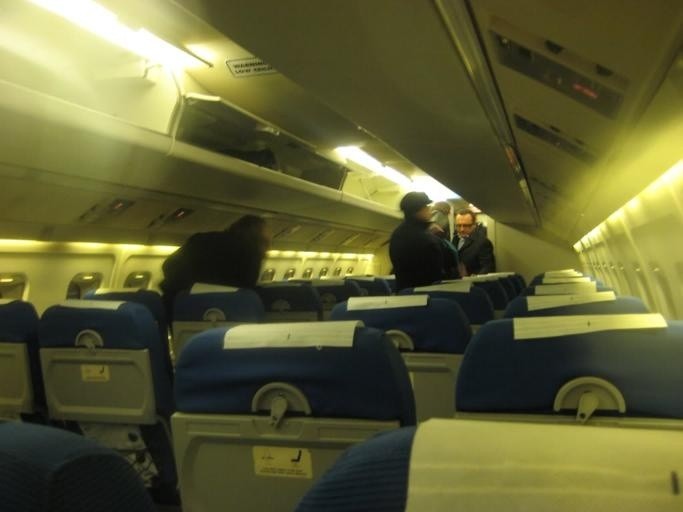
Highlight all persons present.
[425,201,450,241]
[452,207,496,274]
[156,213,272,336]
[387,190,456,289]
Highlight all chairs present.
[0,268,683,512]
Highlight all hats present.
[399,191,435,212]
